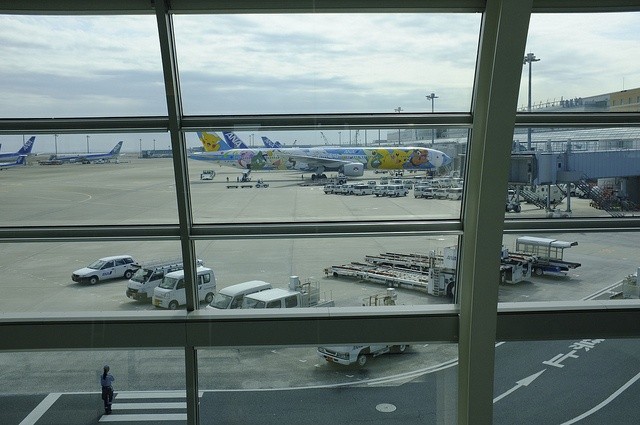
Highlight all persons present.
[100,365,115,414]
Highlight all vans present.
[153,266,216,310]
[206,280,272,310]
[318,344,411,368]
[126,258,204,303]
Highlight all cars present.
[324,176,463,200]
[201,170,215,180]
[72,255,140,285]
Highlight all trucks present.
[324,235,581,300]
[226,177,270,189]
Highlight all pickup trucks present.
[242,288,334,308]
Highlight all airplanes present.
[0,136,36,170]
[188,131,453,180]
[39,141,123,165]
